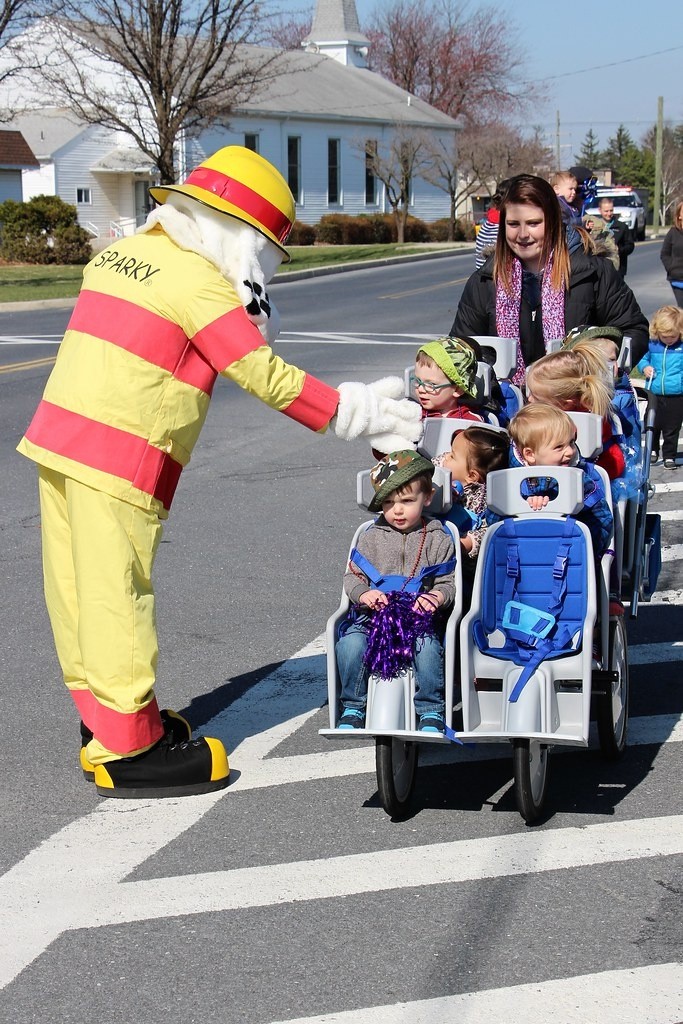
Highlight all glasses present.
[410,375,453,393]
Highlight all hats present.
[569,166,598,186]
[368,449,435,511]
[561,324,623,350]
[417,336,481,398]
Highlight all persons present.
[449,174,650,374]
[335,451,457,730]
[369,337,485,460]
[560,325,642,467]
[599,198,635,279]
[550,171,594,233]
[475,180,509,270]
[527,342,647,615]
[433,403,612,671]
[660,202,683,312]
[458,337,509,428]
[15,145,422,799]
[638,304,682,470]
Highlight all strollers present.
[315,335,661,827]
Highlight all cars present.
[583,186,648,243]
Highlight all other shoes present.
[650,454,658,462]
[664,459,677,469]
[592,644,600,670]
[609,592,624,616]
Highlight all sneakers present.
[336,708,366,729]
[418,712,445,734]
